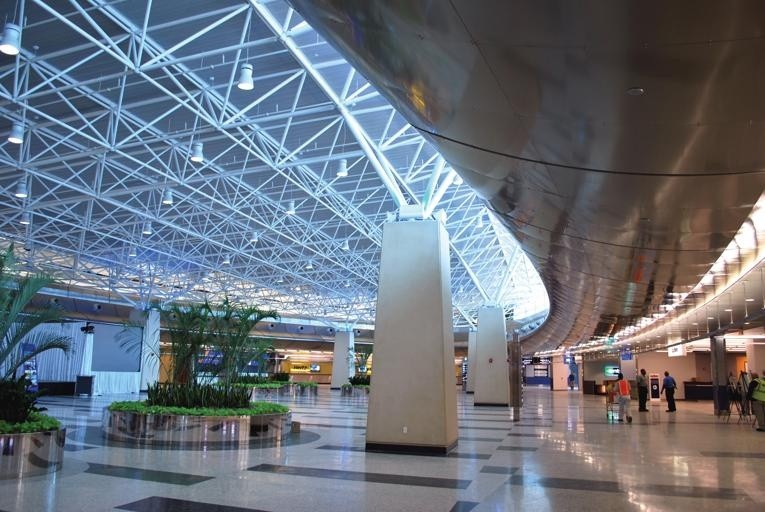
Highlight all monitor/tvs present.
[358,366,367,373]
[564,357,575,364]
[310,364,320,372]
[604,367,619,377]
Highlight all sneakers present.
[755,427,765,432]
[617,419,624,422]
[665,409,677,413]
[627,416,633,424]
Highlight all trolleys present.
[606,390,632,422]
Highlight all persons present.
[568,370,576,391]
[660,370,678,413]
[635,368,650,413]
[728,369,765,432]
[613,373,634,423]
